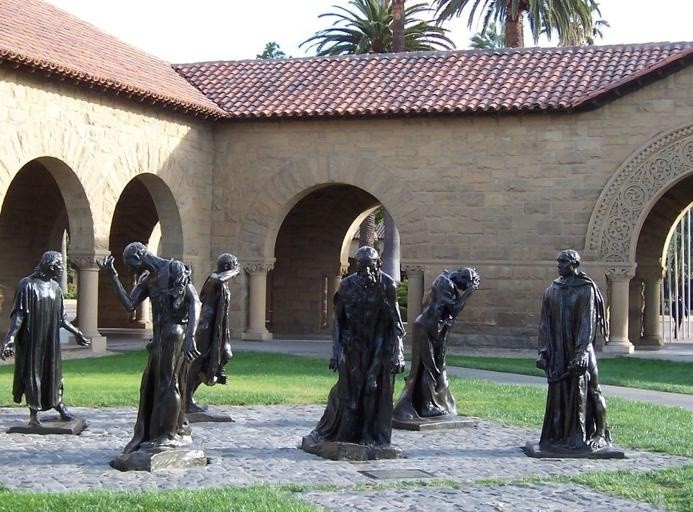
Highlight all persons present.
[1,250,91,427]
[393,267,481,420]
[310,245,406,442]
[96,241,241,453]
[672,295,687,339]
[534,249,613,452]
